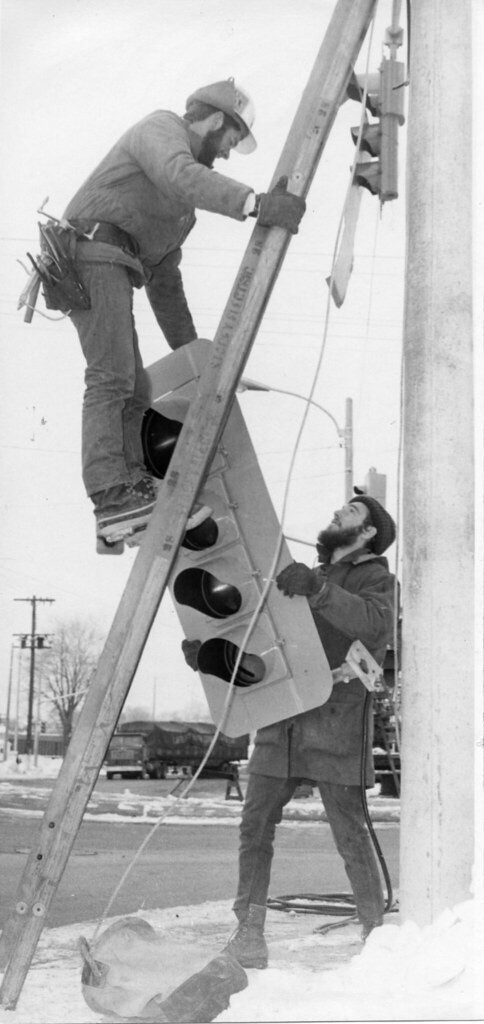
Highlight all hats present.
[348,496,397,555]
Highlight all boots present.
[219,903,268,969]
[361,926,372,943]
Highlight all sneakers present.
[91,476,211,554]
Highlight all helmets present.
[186,78,256,153]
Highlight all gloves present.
[256,176,308,233]
[275,563,324,599]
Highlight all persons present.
[59,81,305,555]
[220,497,396,968]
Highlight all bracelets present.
[249,194,259,217]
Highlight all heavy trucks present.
[102,721,250,783]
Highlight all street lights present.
[235,378,354,506]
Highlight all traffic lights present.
[350,56,401,201]
[132,336,334,736]
[41,721,45,734]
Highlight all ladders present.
[1,0,398,1014]
